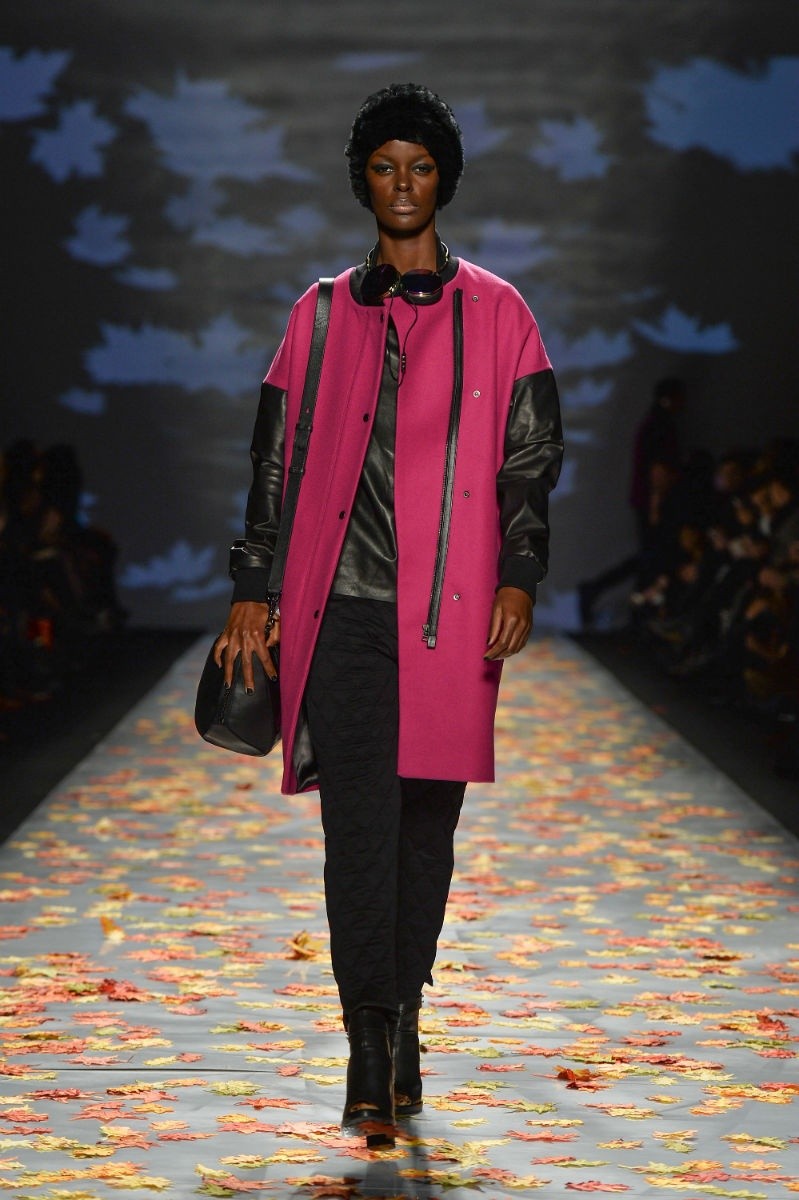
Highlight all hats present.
[344,82,464,213]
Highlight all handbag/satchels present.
[195,591,284,758]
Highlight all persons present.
[214,77,564,1150]
[0,437,132,749]
[581,378,798,783]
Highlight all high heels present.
[342,1001,400,1153]
[393,1027,424,1115]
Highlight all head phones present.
[361,240,450,305]
